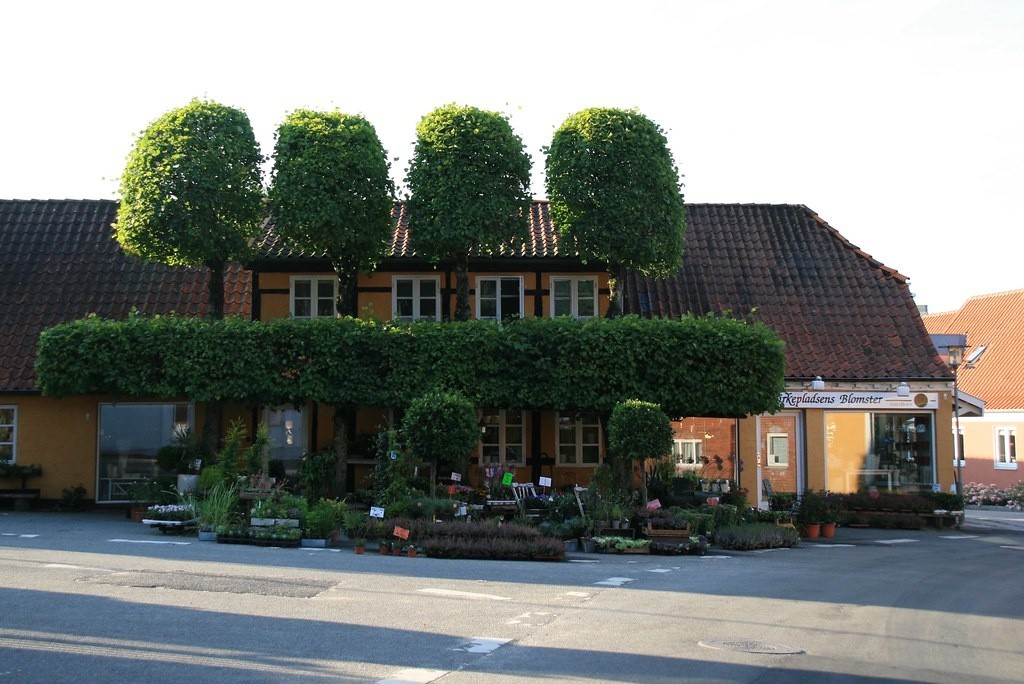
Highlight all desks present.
[842,468,900,493]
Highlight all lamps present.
[806,376,824,390]
[891,382,909,398]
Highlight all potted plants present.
[132,425,346,547]
[950,495,964,516]
[822,506,838,537]
[407,544,417,556]
[393,543,403,555]
[929,491,948,515]
[380,539,389,555]
[526,489,712,556]
[355,537,367,554]
[802,491,822,538]
